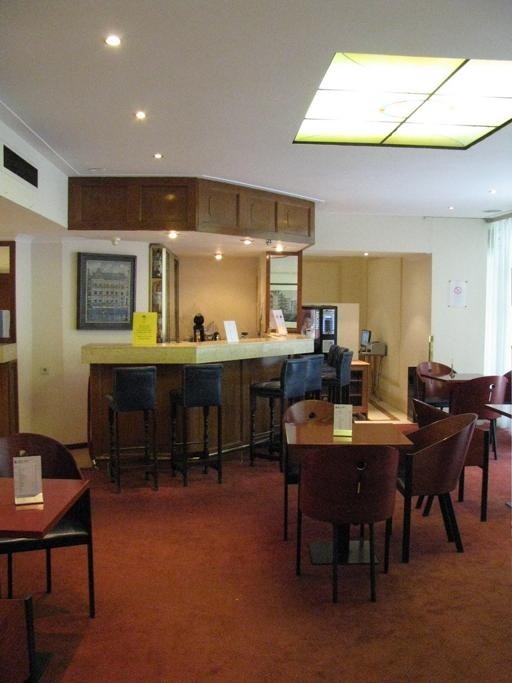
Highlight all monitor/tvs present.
[360,329,371,353]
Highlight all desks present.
[0,476,92,539]
[284,421,413,565]
[420,372,487,382]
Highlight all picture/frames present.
[77,251,137,330]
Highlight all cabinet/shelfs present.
[350,360,371,420]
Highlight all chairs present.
[395,413,479,563]
[0,431,95,618]
[249,356,310,472]
[103,365,158,490]
[323,346,349,402]
[416,361,457,410]
[321,351,354,405]
[411,396,490,521]
[490,370,512,451]
[0,596,53,682]
[296,443,398,602]
[270,354,324,407]
[324,343,340,401]
[281,399,372,541]
[449,375,509,460]
[169,363,223,486]
